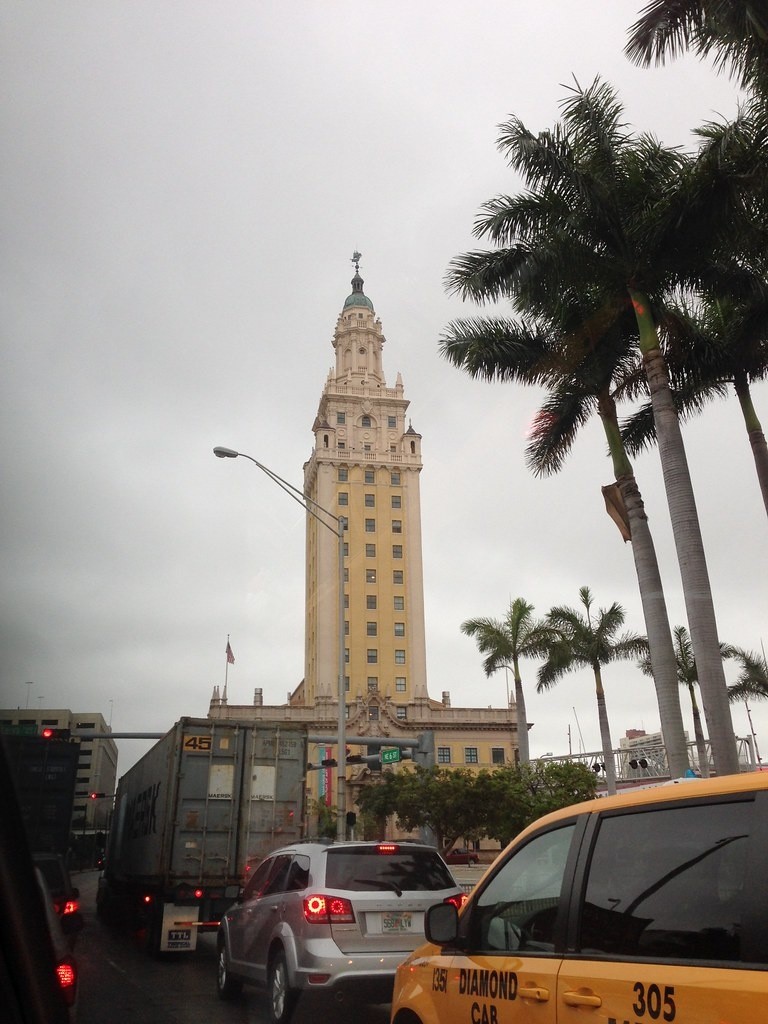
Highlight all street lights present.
[212,445,354,843]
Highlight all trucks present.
[97,714,310,944]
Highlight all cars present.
[32,849,80,930]
[97,851,106,871]
[215,838,472,1022]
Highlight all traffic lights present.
[601,763,606,771]
[592,765,598,770]
[638,758,647,768]
[629,760,638,770]
[89,792,105,799]
[41,727,70,741]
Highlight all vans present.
[391,765,767,1023]
